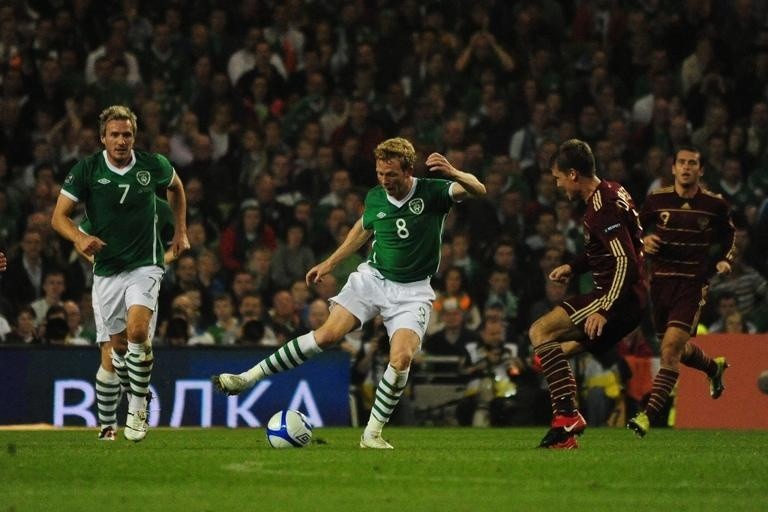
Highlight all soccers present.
[268,410,313,448]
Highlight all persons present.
[218,136,486,451]
[73,198,180,442]
[624,142,740,440]
[527,138,653,451]
[1,1,768,429]
[50,106,192,442]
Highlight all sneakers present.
[706,357,729,399]
[626,412,650,439]
[215,373,254,394]
[537,437,579,450]
[124,400,147,443]
[98,423,118,441]
[359,433,394,449]
[540,409,587,447]
